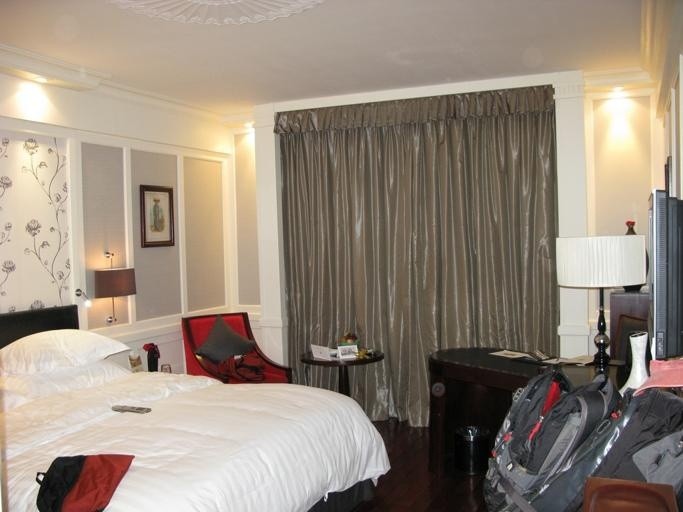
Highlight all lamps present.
[554,234,647,367]
[74,251,137,323]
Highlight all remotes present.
[112,405,151,413]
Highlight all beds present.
[0,303,373,512]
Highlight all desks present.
[427,346,552,477]
[298,352,384,397]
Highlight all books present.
[563,354,594,365]
[523,348,550,362]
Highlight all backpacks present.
[483,366,682,511]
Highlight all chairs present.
[180,311,292,384]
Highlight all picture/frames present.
[139,184,174,248]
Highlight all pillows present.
[191,313,255,365]
[0,327,132,395]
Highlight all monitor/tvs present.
[648,189,683,360]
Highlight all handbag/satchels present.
[219,353,266,382]
[35,454,134,512]
